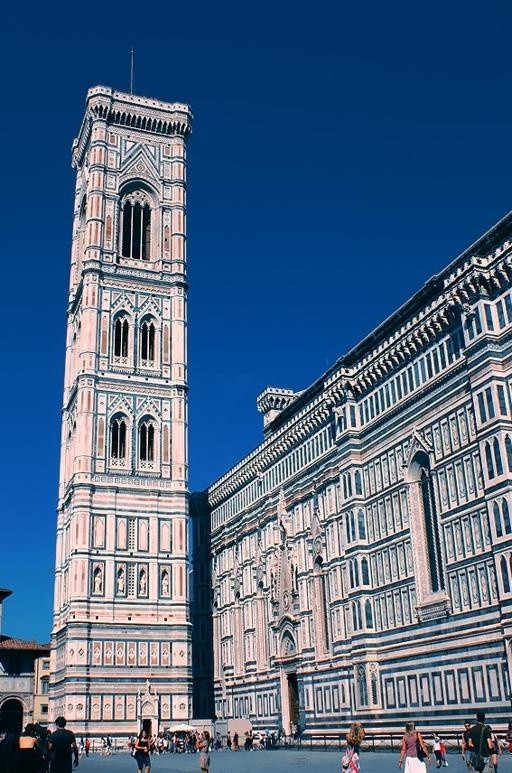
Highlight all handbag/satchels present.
[473,754,484,770]
[131,749,137,756]
[197,743,215,752]
[416,739,428,757]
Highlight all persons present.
[135,730,152,773]
[398,711,512,772]
[195,729,211,773]
[0,716,80,772]
[105,733,113,756]
[99,736,107,755]
[78,735,84,758]
[342,721,365,772]
[85,738,91,757]
[127,720,298,753]
